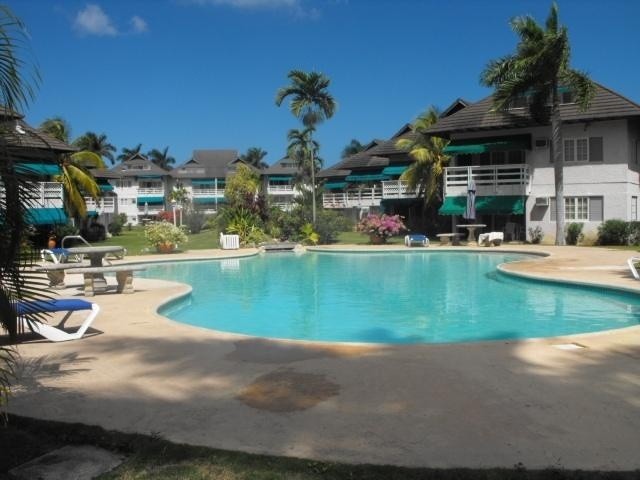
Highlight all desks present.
[456,224,487,243]
[66,247,127,293]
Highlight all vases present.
[156,244,175,254]
[371,235,386,244]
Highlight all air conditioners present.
[535,138,548,150]
[535,196,549,208]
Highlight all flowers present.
[142,215,189,252]
[356,211,410,240]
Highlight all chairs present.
[43,248,84,262]
[5,297,97,341]
[404,232,429,247]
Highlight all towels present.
[479,232,503,244]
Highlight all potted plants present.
[528,226,542,243]
[46,231,58,250]
[565,221,584,246]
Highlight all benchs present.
[67,264,148,295]
[34,261,111,288]
[438,233,465,245]
[479,230,504,248]
